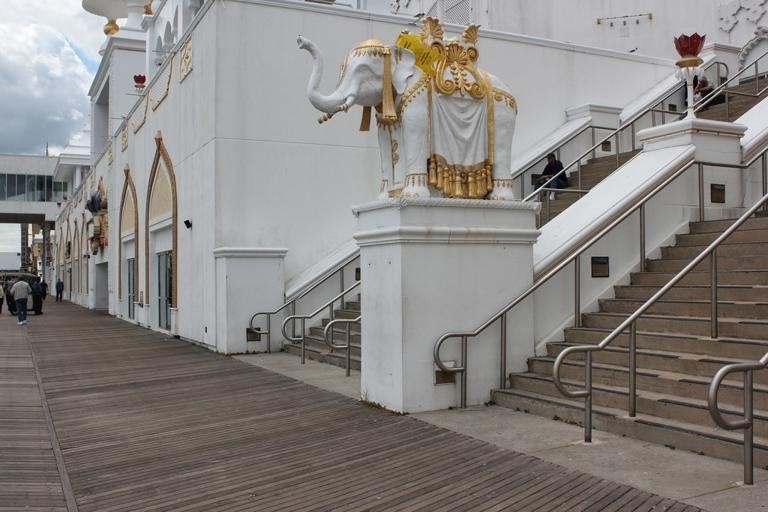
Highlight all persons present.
[693,76,717,110]
[531,152,570,202]
[0,276,64,325]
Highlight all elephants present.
[297,36,518,201]
